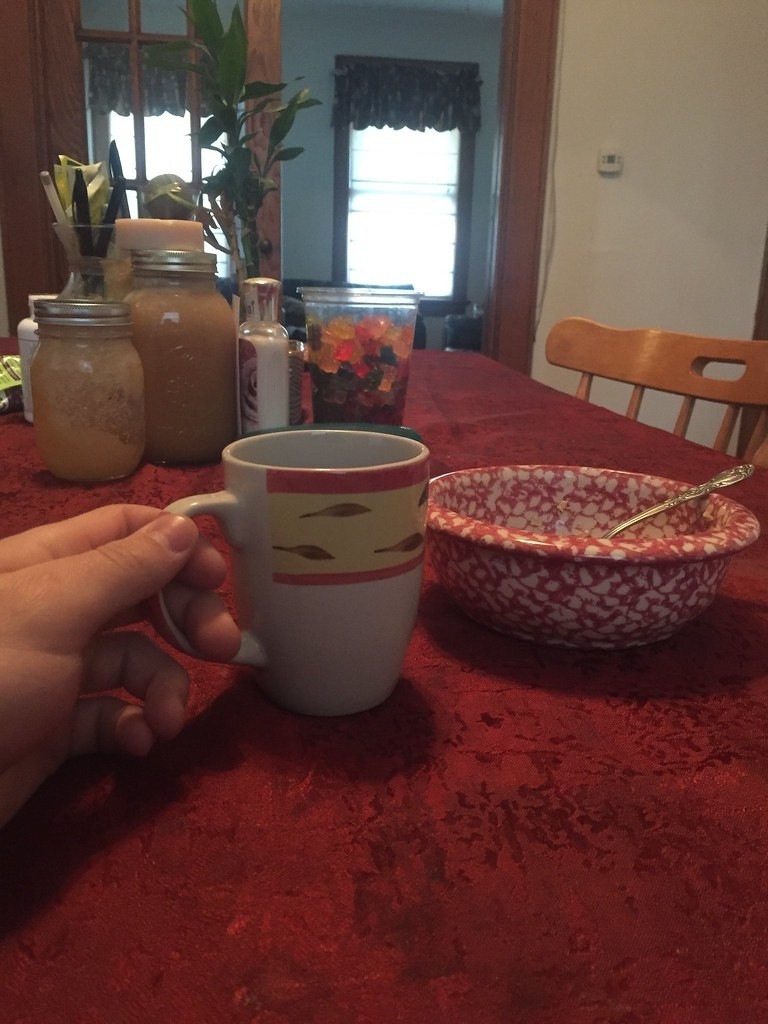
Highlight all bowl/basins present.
[428,466,761,646]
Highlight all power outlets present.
[597,147,623,173]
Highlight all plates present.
[237,421,422,446]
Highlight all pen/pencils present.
[39,140,130,258]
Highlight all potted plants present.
[141,1,325,427]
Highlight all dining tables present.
[1,336,768,1022]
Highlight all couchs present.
[281,278,426,350]
[441,313,482,352]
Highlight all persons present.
[0,501,241,831]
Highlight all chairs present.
[544,316,768,470]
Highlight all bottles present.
[17,294,59,425]
[109,219,204,297]
[235,275,290,433]
[53,221,116,296]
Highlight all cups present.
[288,340,306,426]
[142,429,430,719]
[298,284,421,426]
